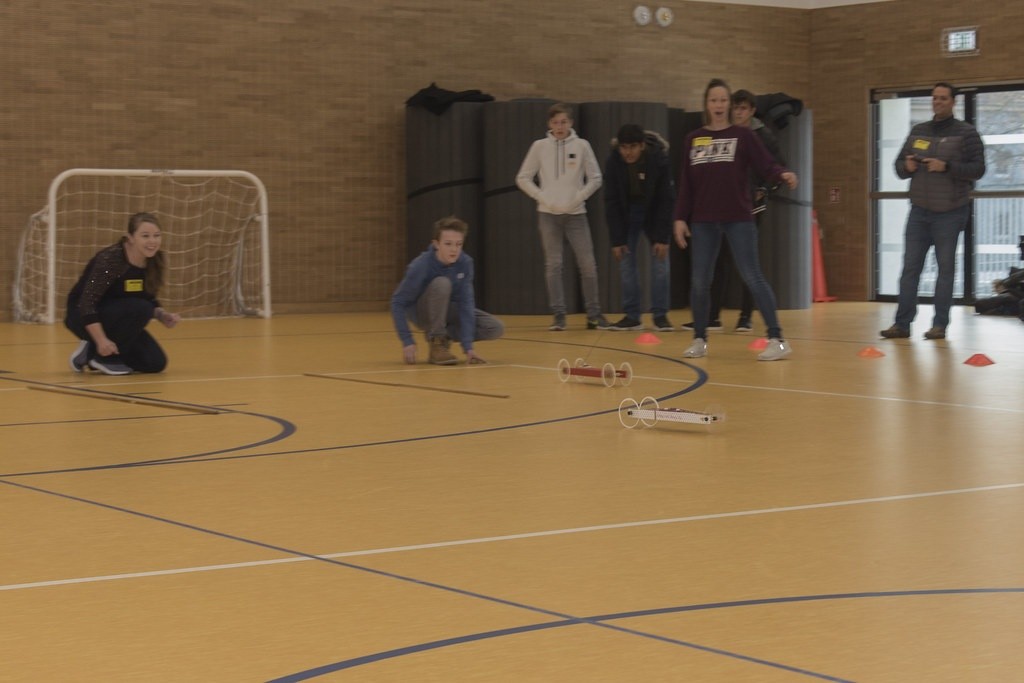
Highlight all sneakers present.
[736,317,753,332]
[924,323,945,339]
[430,335,459,364]
[611,315,644,330]
[550,310,566,331]
[683,337,709,359]
[757,337,791,360]
[68,339,90,372]
[654,315,675,331]
[879,321,911,338]
[588,313,611,330]
[682,318,722,331]
[89,357,131,375]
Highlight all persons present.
[681,88,798,332]
[391,218,504,364]
[602,124,673,331]
[674,78,792,361]
[516,102,612,330]
[64,213,179,377]
[879,82,984,339]
